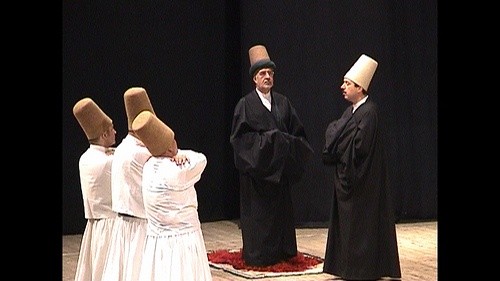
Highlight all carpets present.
[207,247,324,278]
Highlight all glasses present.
[256,71,274,78]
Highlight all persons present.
[73,98,116,281]
[323,54,402,281]
[131,110,212,281]
[230,45,314,269]
[100,87,156,281]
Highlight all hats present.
[124,88,157,131]
[344,54,378,91]
[73,98,113,140]
[131,111,174,156]
[249,45,275,77]
